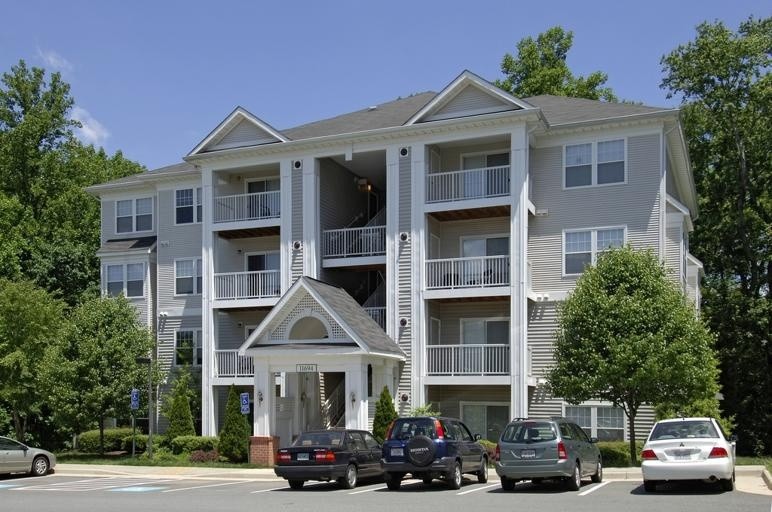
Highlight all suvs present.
[422,451,425,453]
[492,415,603,491]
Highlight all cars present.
[274,427,385,490]
[380,415,489,491]
[641,416,739,492]
[0,435,56,482]
